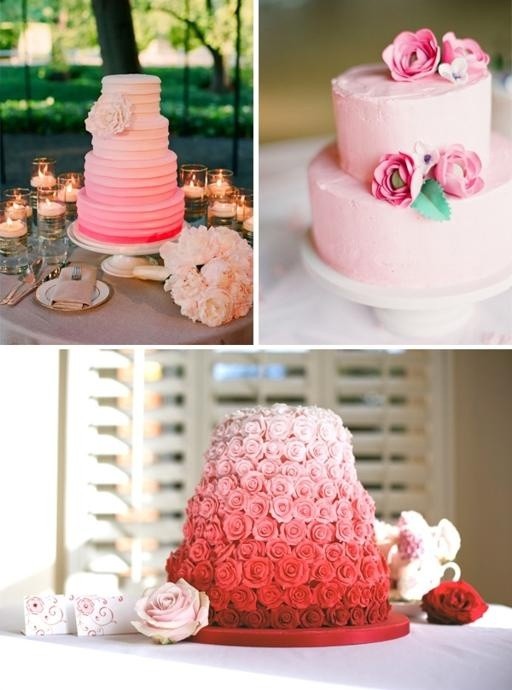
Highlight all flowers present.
[131,578,211,645]
[419,580,488,626]
[370,27,490,221]
[134,225,253,328]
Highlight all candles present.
[0,165,253,238]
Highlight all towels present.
[49,265,97,311]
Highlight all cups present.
[391,558,461,602]
[0,157,84,274]
[179,163,253,241]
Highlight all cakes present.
[309,28,512,288]
[129,402,392,644]
[73,74,185,250]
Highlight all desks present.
[0,602,512,690]
[0,214,252,345]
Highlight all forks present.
[72,265,82,280]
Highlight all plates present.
[35,275,115,313]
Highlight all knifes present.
[0,256,63,306]
